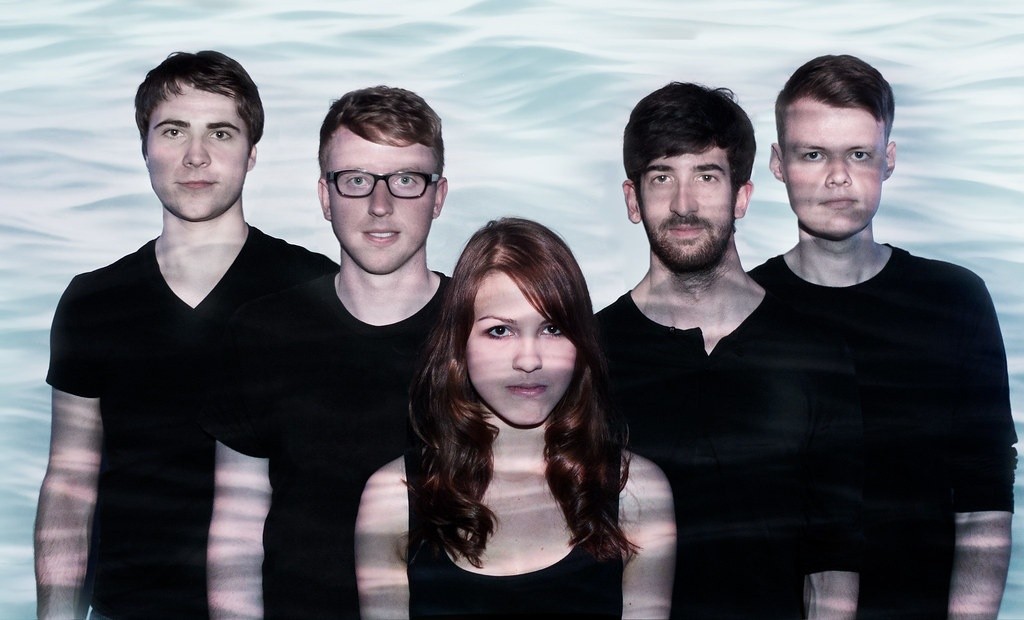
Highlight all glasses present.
[326,168,440,199]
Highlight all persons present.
[590,82,864,620]
[30,50,342,619]
[352,214,678,620]
[740,53,1018,620]
[204,84,457,619]
